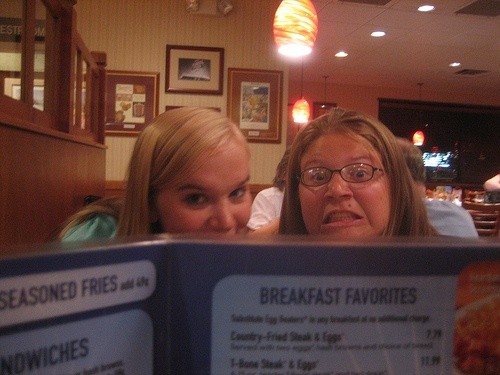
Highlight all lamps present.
[185,0,233,17]
[412,83,425,146]
[291,55,310,124]
[272,0,319,57]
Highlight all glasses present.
[295,162,388,187]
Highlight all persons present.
[47,106,252,243]
[277,107,440,237]
[484,173,500,192]
[397,138,479,236]
[246,145,292,238]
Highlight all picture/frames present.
[226,67,283,144]
[164,44,225,97]
[165,106,220,113]
[104,70,160,137]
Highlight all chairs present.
[461,201,500,238]
